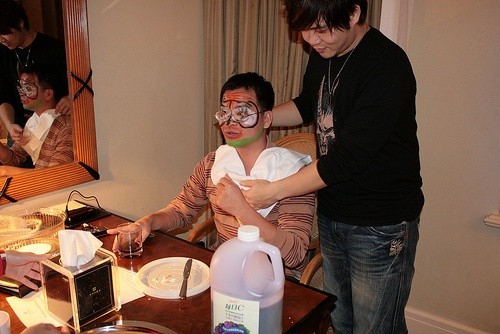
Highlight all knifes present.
[178,258,192,299]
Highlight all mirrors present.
[0,0,100,205]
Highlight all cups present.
[0,310,11,334]
[116,222,144,259]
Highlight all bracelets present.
[0,250,6,275]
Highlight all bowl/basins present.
[0,207,67,251]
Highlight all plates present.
[78,320,178,334]
[2,235,60,272]
[134,256,211,300]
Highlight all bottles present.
[209,224,286,334]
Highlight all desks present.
[0,199,338,334]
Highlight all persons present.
[0,248,70,334]
[0,1,71,146]
[107,71,315,284]
[0,70,76,172]
[264,0,426,334]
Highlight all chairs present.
[186,132,324,291]
